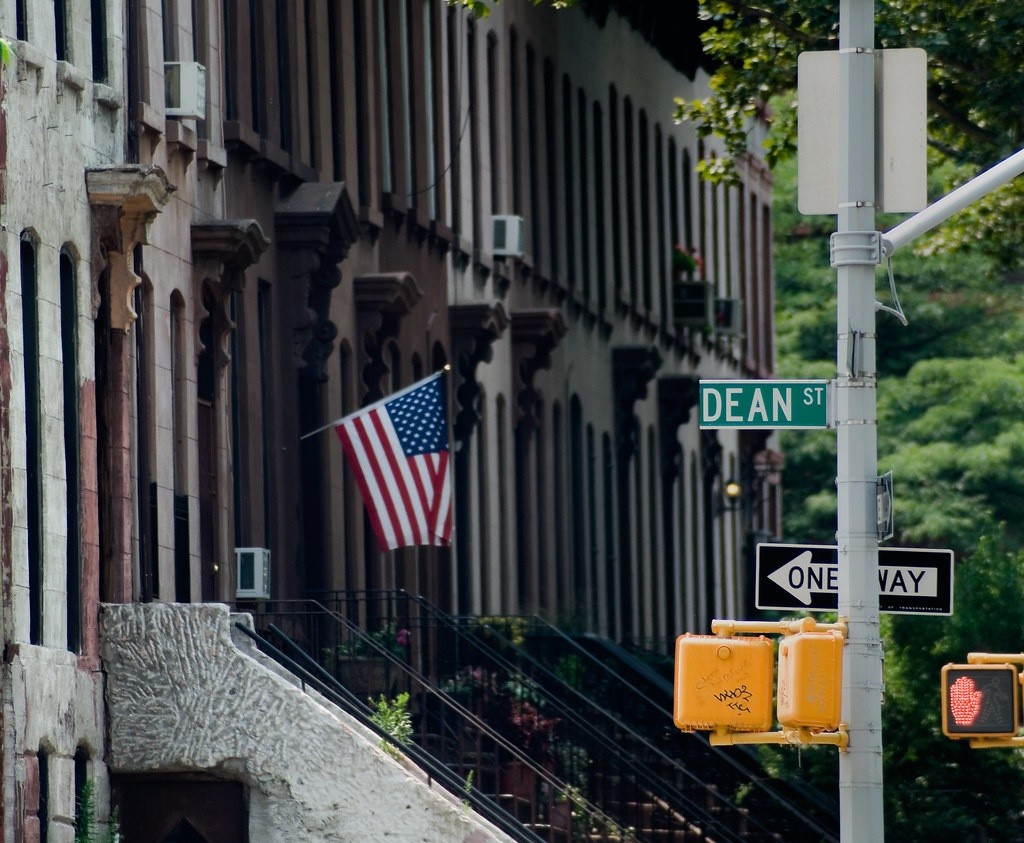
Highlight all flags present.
[334,369,457,552]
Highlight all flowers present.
[323,622,411,678]
[503,695,561,759]
[428,660,499,703]
[541,781,589,843]
[673,245,701,273]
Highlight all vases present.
[337,652,398,699]
[550,801,569,831]
[419,692,467,743]
[502,759,536,799]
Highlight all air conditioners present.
[163,61,206,119]
[494,214,524,256]
[674,281,717,329]
[233,548,271,600]
[714,299,743,333]
